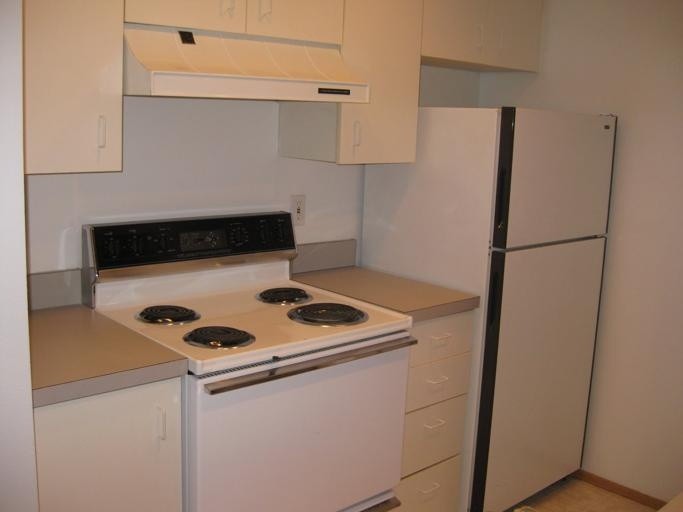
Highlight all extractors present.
[119,25,370,104]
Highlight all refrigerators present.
[364,103,616,512]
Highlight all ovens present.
[178,344,415,512]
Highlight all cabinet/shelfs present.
[423,4,543,79]
[122,2,341,52]
[392,308,480,512]
[23,0,125,173]
[33,376,184,512]
[344,3,421,165]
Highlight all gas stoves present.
[104,280,409,371]
